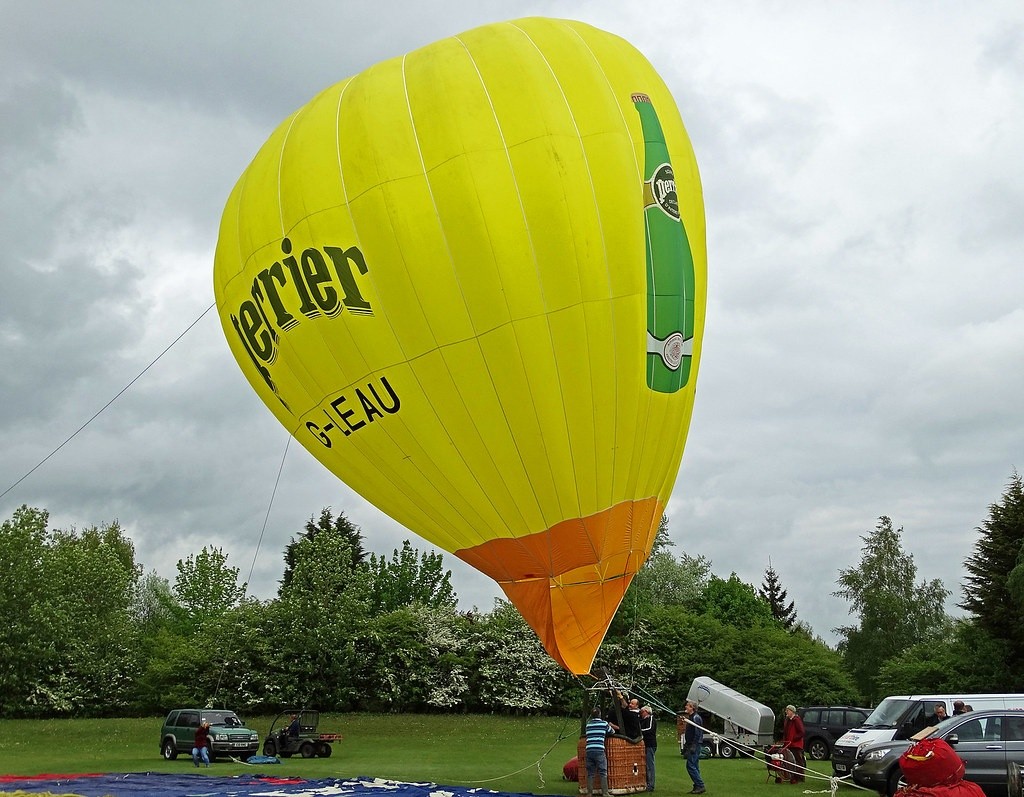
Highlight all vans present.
[831,694,1024,775]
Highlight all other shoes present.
[587,793,592,797]
[603,793,614,797]
[797,776,805,782]
[691,785,706,794]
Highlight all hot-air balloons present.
[210,16,708,797]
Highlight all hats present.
[787,705,798,714]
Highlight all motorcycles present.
[263,710,343,759]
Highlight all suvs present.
[851,710,1024,797]
[159,709,259,762]
[797,706,875,761]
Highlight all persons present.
[641,705,657,791]
[192,720,209,768]
[585,707,615,797]
[932,701,983,739]
[279,714,300,749]
[685,701,706,793]
[608,689,642,740]
[783,705,807,782]
